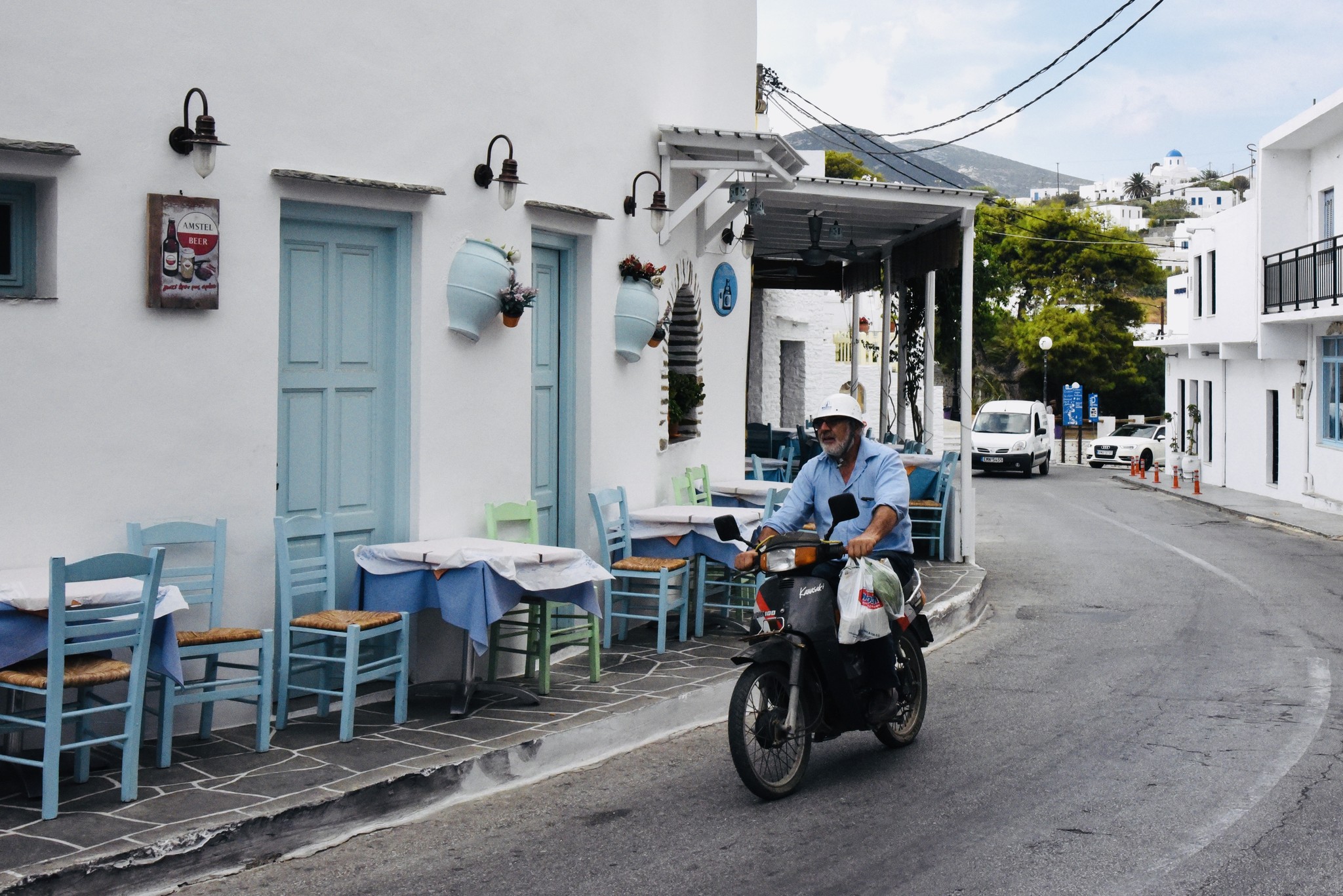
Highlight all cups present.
[181,247,196,283]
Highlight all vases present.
[647,331,665,348]
[448,237,518,344]
[616,276,658,361]
[859,323,870,335]
[502,311,523,328]
[890,321,895,332]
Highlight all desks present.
[0,567,191,780]
[614,506,776,638]
[770,427,814,458]
[349,537,617,724]
[882,444,905,454]
[744,456,788,483]
[898,453,943,499]
[694,479,814,525]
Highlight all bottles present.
[163,218,180,276]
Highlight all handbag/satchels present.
[837,556,891,644]
[855,557,905,620]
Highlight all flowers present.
[859,316,870,324]
[655,300,676,330]
[619,255,667,292]
[484,236,521,267]
[499,273,539,315]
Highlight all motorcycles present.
[714,493,936,799]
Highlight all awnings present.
[747,167,986,562]
[658,120,805,254]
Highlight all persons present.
[733,393,914,725]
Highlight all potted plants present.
[668,370,706,438]
[1181,404,1201,479]
[1163,411,1185,476]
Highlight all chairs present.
[485,500,601,694]
[588,485,690,654]
[670,420,960,638]
[125,518,274,769]
[0,546,167,821]
[272,510,410,742]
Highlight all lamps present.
[623,170,675,235]
[746,172,766,218]
[170,86,230,182]
[722,206,762,260]
[474,134,528,211]
[726,149,750,204]
[846,227,858,259]
[827,208,843,242]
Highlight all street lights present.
[1039,336,1052,412]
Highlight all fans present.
[754,209,881,267]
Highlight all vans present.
[970,400,1052,479]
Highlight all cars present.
[1085,423,1167,472]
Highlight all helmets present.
[812,393,864,428]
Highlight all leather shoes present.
[868,688,899,725]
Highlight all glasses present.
[813,416,853,429]
